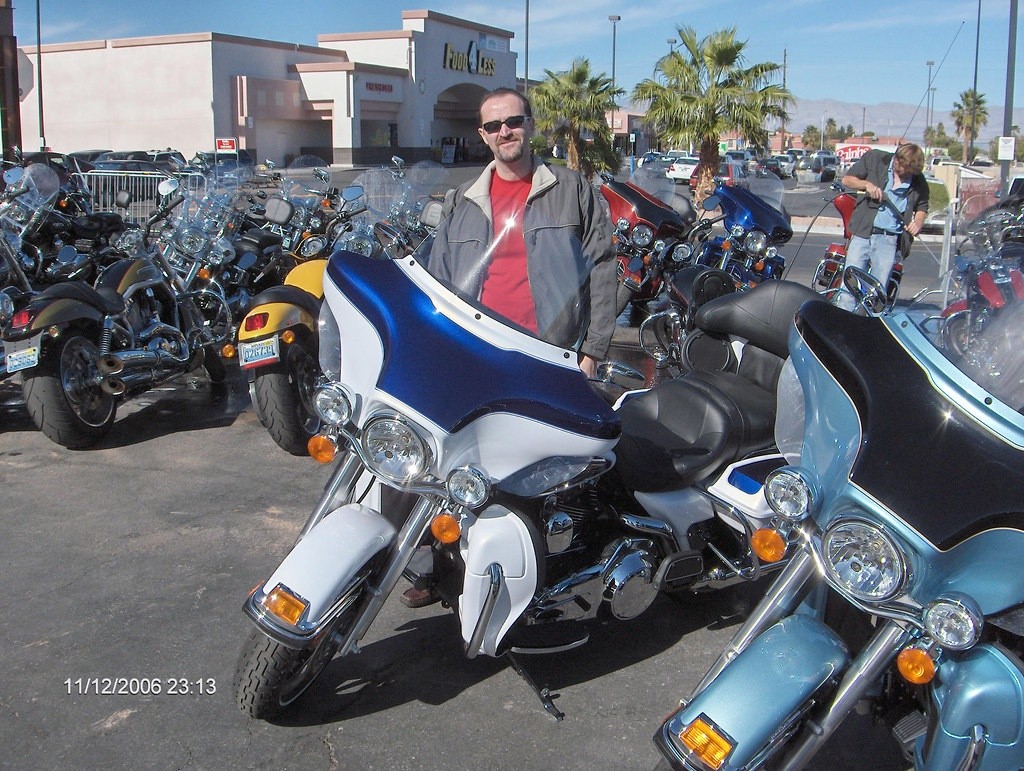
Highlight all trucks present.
[810,149,837,167]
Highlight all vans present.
[786,148,805,163]
[189,148,254,177]
[667,150,689,159]
[725,150,752,167]
[772,155,797,178]
[689,163,751,197]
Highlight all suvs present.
[932,156,950,167]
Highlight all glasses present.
[484,116,530,134]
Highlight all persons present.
[400,86,618,608]
[836,142,930,312]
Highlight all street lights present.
[609,14,621,149]
[926,61,936,134]
[667,39,676,55]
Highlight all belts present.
[871,227,895,235]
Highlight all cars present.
[637,152,663,167]
[69,147,185,199]
[755,160,785,178]
[0,150,100,206]
[667,156,701,184]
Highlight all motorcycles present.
[918,172,1024,382]
[0,153,795,451]
[812,182,903,316]
[231,249,939,722]
[651,266,1024,771]
